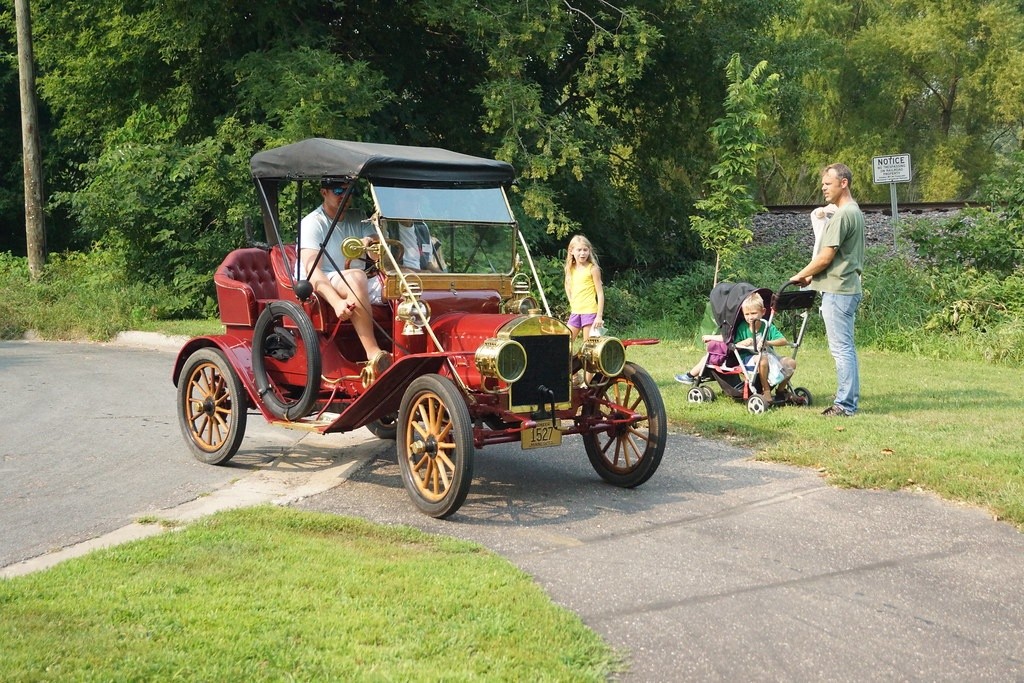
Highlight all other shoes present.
[572,376,584,388]
[366,350,394,379]
[580,381,589,389]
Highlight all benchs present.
[214,247,279,327]
[270,245,392,333]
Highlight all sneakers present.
[820,405,857,417]
[672,370,694,385]
[763,390,773,403]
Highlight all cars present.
[170,136,668,519]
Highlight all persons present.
[789,163,865,415]
[674,335,725,385]
[809,202,839,258]
[380,216,444,274]
[564,235,604,388]
[296,175,416,380]
[733,292,803,405]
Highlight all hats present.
[321,174,353,189]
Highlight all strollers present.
[687,277,817,416]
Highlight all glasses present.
[322,186,355,195]
